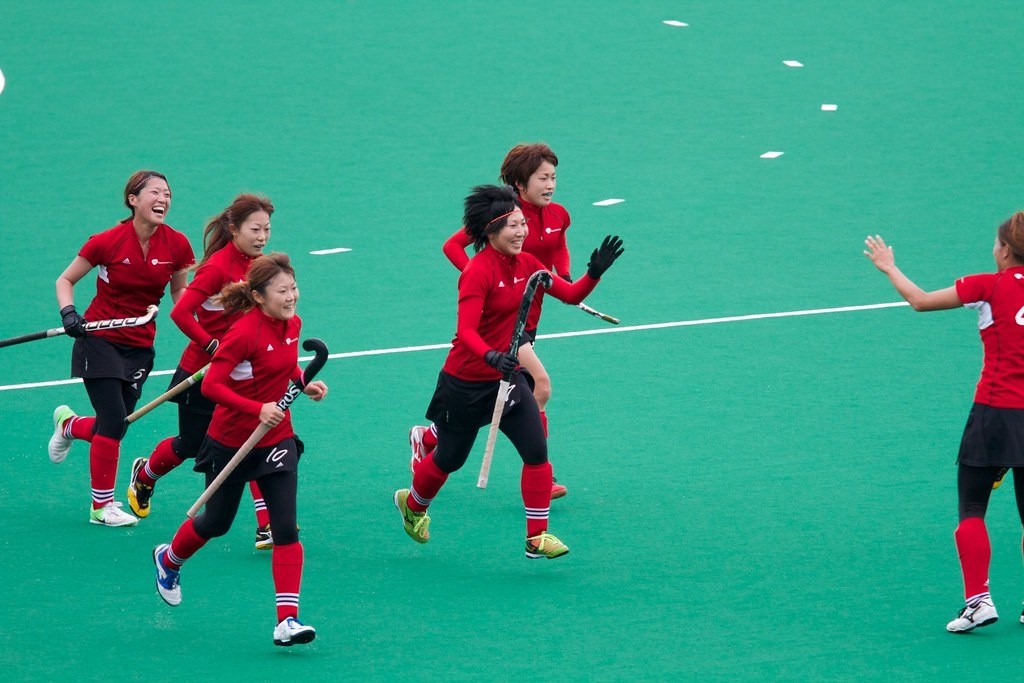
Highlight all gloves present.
[484,350,520,374]
[586,235,625,279]
[60,305,88,338]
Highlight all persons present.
[48,170,194,528]
[861,212,1024,634]
[152,254,329,646]
[409,143,569,498]
[393,186,625,558]
[127,193,301,547]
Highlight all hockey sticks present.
[577,301,621,326]
[185,338,329,519]
[123,360,212,426]
[476,270,553,489]
[0,304,159,350]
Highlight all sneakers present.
[129,457,151,517]
[89,501,137,526]
[255,525,300,550]
[410,426,430,474]
[48,406,75,463]
[153,543,181,607]
[526,530,569,558]
[393,489,429,543]
[550,476,567,500]
[946,597,998,632]
[273,616,316,646]
